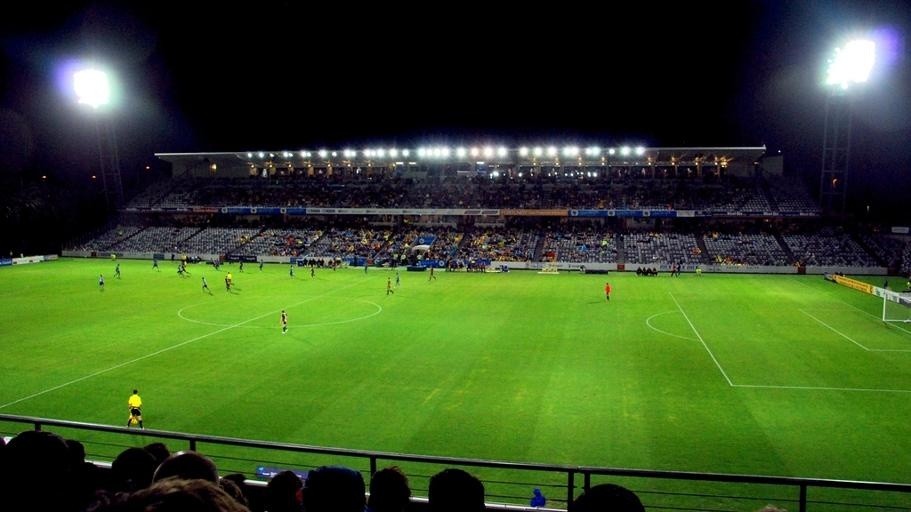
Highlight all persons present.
[279,310,289,334]
[10,171,910,301]
[1,430,486,511]
[125,389,145,430]
[761,504,788,512]
[530,487,546,508]
[567,483,646,512]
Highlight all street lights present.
[74,68,124,229]
[820,39,877,211]
